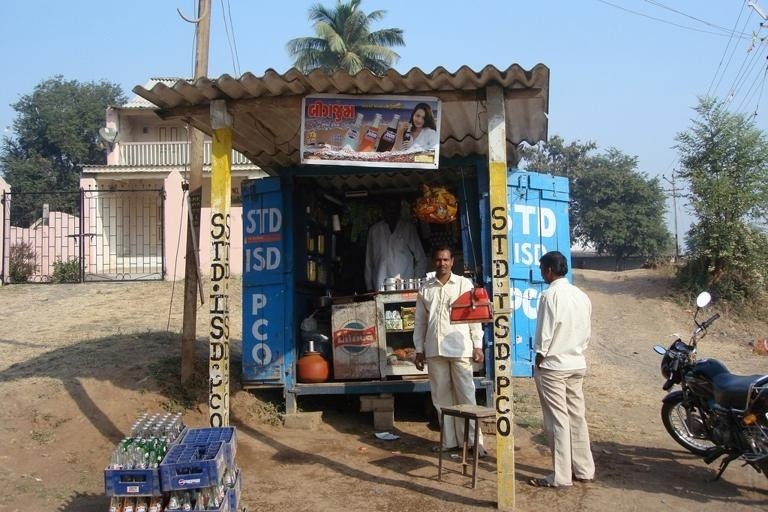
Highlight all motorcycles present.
[653,291,768,486]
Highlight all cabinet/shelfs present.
[295,186,351,293]
[376,292,483,380]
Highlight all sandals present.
[529,477,572,488]
[432,444,487,459]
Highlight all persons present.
[394,102,436,151]
[364,194,428,292]
[527,251,597,487]
[410,242,490,458]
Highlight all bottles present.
[109,470,236,512]
[375,113,401,152]
[384,277,422,291]
[403,124,413,141]
[342,113,365,150]
[110,410,183,470]
[357,113,383,151]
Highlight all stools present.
[437,405,496,489]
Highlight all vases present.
[297,351,328,382]
